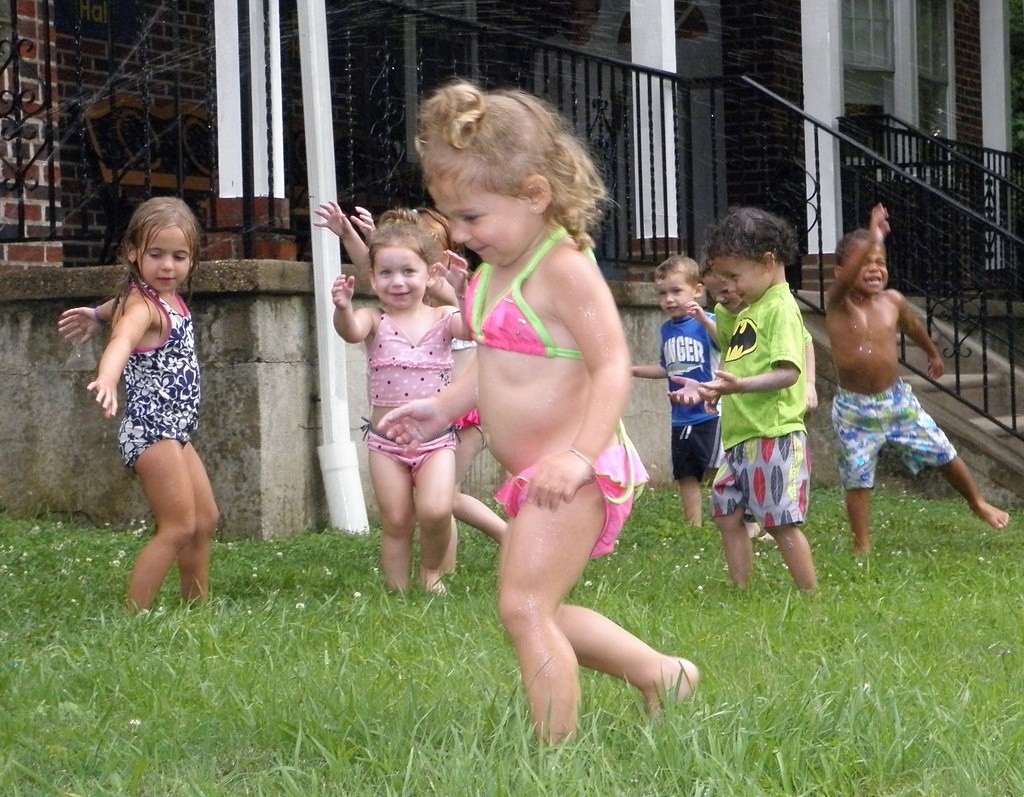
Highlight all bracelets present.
[570,449,598,485]
[95,306,110,325]
[454,293,466,299]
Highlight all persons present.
[829,201,1012,556]
[314,78,819,746]
[57,196,221,616]
